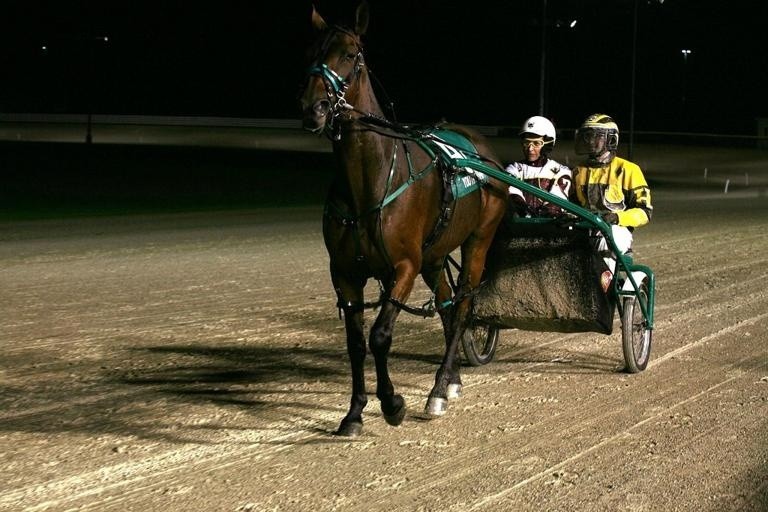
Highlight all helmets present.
[574,113,620,165]
[518,115,556,147]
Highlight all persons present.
[506,114,573,220]
[573,113,653,275]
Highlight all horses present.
[298,3,510,438]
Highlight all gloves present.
[605,213,618,225]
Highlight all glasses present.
[522,138,544,148]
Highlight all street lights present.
[680,50,691,104]
[539,20,577,117]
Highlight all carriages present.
[298,2,656,437]
